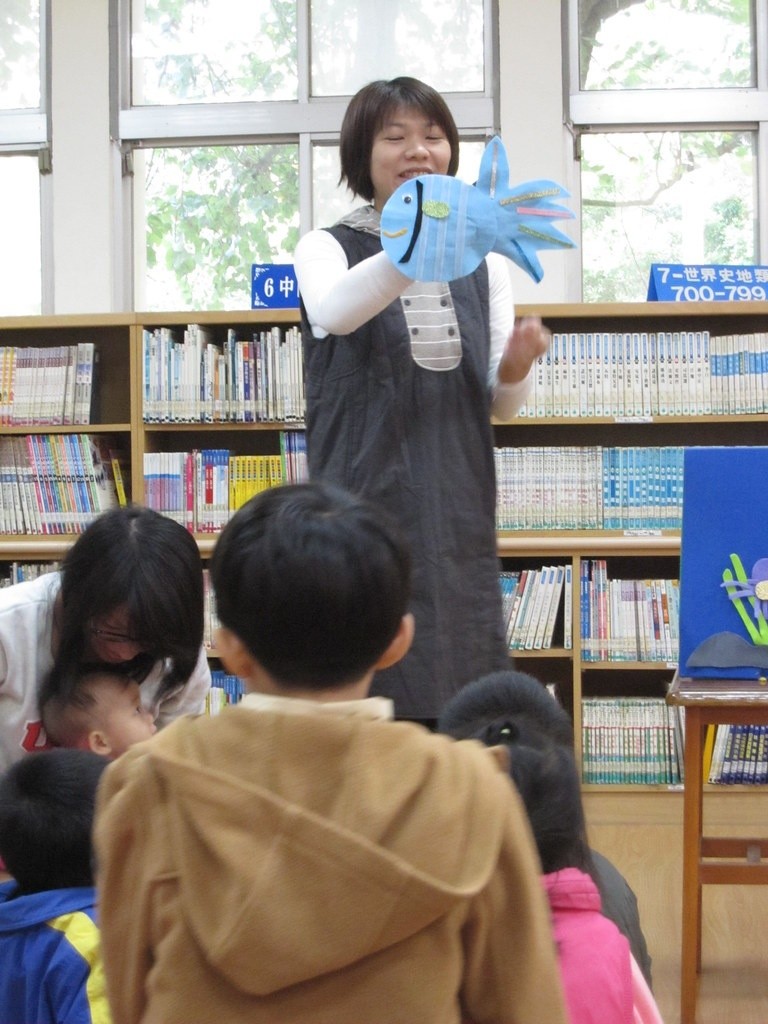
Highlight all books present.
[516,333,768,418]
[202,569,246,716]
[0,342,129,535]
[495,559,681,663]
[492,445,685,530]
[580,696,768,786]
[0,562,62,589]
[143,323,311,533]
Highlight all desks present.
[663,663,768,1024]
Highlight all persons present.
[0,749,120,1024]
[0,504,213,778]
[437,670,666,1024]
[37,650,158,763]
[294,77,554,734]
[89,480,575,1024]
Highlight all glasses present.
[89,617,142,642]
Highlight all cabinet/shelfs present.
[0,294,768,793]
